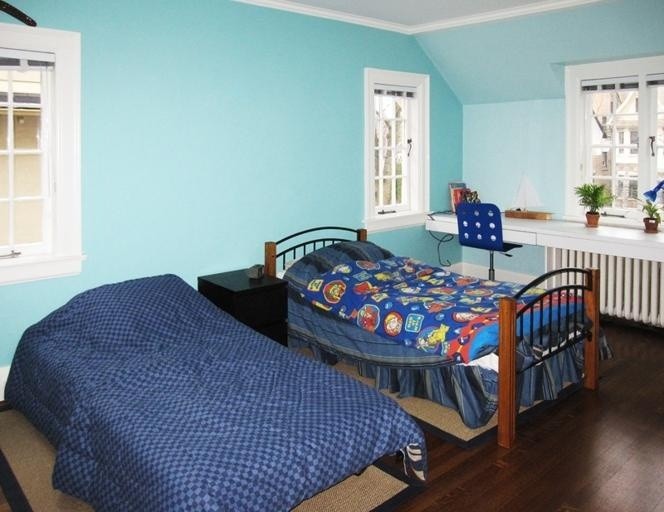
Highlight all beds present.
[261,226,601,446]
[24,271,425,510]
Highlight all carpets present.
[1,406,427,510]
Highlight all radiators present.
[545,249,663,331]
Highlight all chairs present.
[456,204,523,282]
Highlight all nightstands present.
[197,265,290,352]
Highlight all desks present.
[426,210,662,258]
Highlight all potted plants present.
[573,184,660,234]
